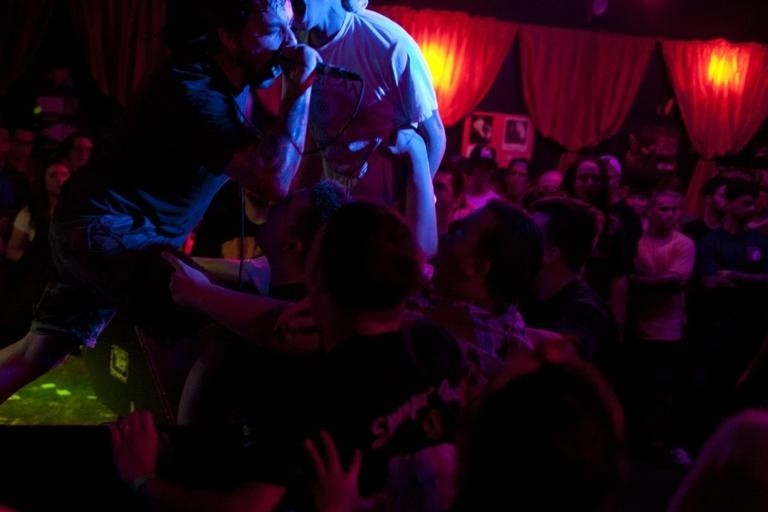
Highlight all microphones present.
[268,48,360,88]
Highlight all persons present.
[0,3,768,512]
